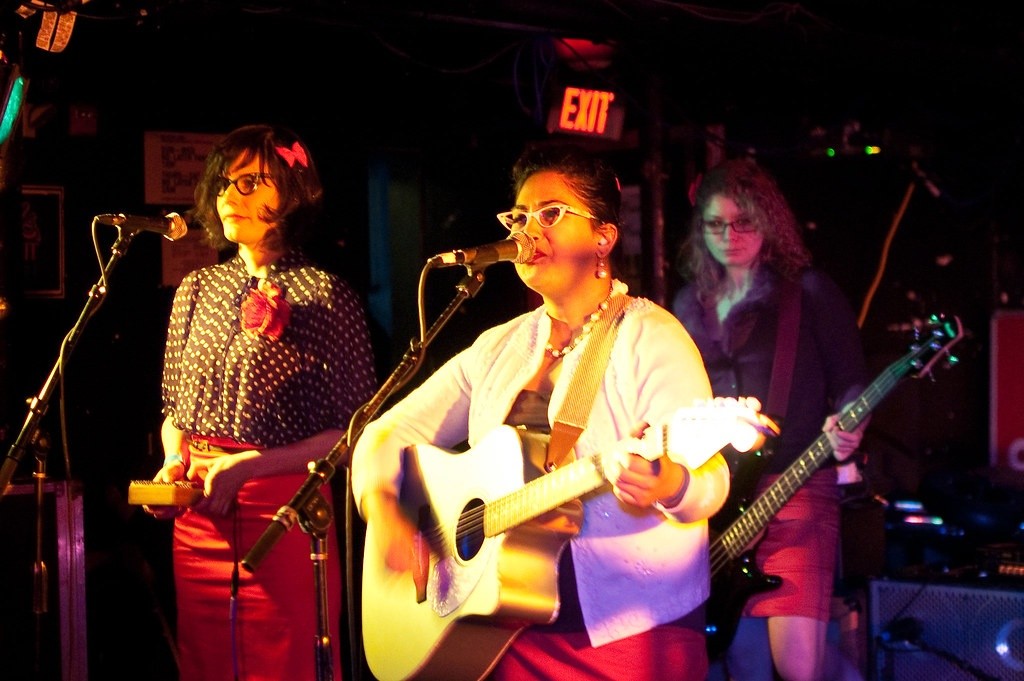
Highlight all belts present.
[188,439,246,452]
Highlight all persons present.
[141,125,379,681]
[348,143,730,680]
[671,159,871,681]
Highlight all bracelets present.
[164,455,186,466]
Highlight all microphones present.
[95,211,188,241]
[877,617,920,643]
[910,162,940,197]
[427,231,536,269]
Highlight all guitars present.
[357,394,782,681]
[705,308,969,668]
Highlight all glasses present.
[497,204,606,232]
[213,173,281,197]
[699,218,758,234]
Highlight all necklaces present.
[545,285,615,358]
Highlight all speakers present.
[866,571,1024,681]
